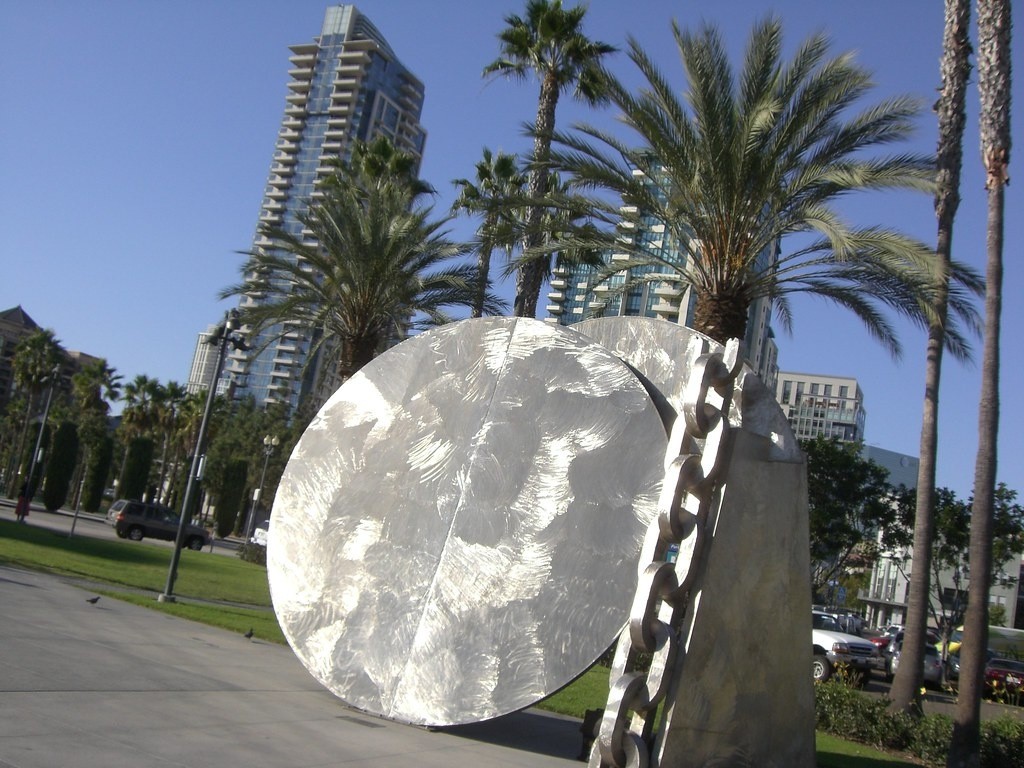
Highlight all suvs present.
[104,498,211,552]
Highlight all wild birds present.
[243,624,256,641]
[85,594,102,607]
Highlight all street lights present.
[157,311,257,599]
[18,363,62,522]
[242,433,281,546]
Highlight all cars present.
[250,520,269,545]
[811,605,1024,700]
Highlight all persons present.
[889,629,905,652]
[14,475,33,523]
